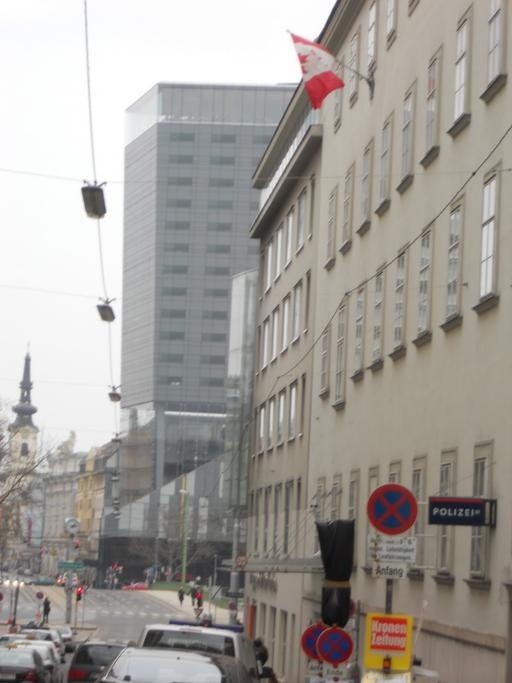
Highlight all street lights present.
[1,578,24,631]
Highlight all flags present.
[290,31,344,109]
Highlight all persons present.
[43,596,52,622]
[186,585,199,606]
[177,585,188,605]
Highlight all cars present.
[0,619,261,682]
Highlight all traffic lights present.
[197,591,203,606]
[76,585,83,600]
